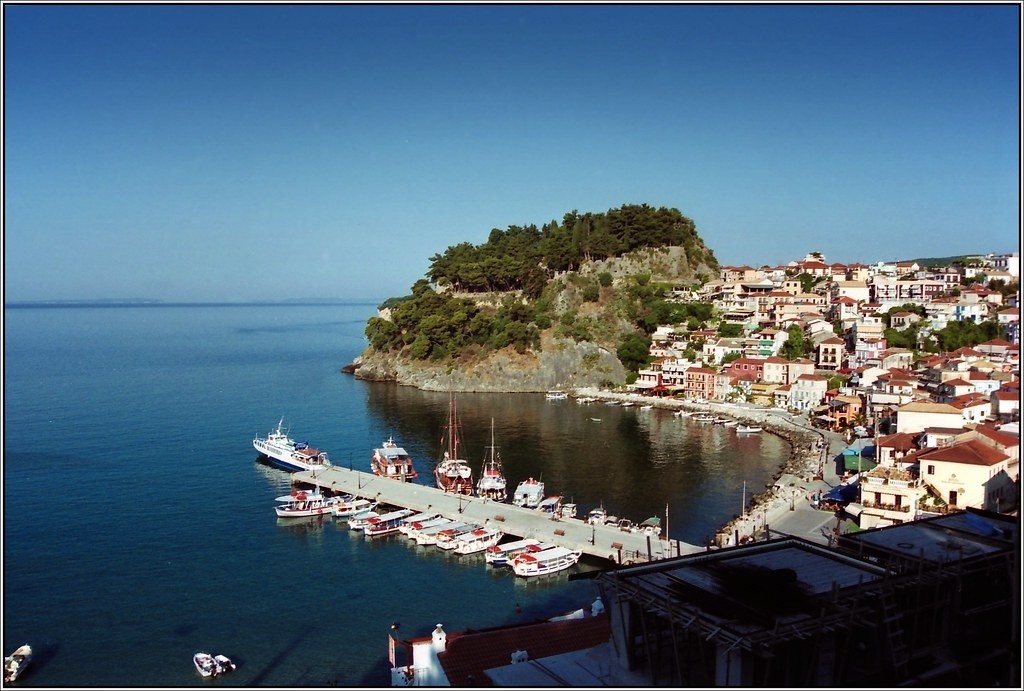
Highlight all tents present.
[823,484,858,502]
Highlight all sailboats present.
[433,389,474,497]
[475,416,508,502]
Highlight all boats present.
[622,401,634,407]
[640,404,654,411]
[673,409,763,434]
[273,481,584,577]
[561,503,578,519]
[588,499,608,526]
[577,393,596,403]
[546,390,569,401]
[510,477,545,508]
[191,653,236,679]
[369,435,417,481]
[252,414,329,472]
[535,495,562,518]
[4,643,34,683]
[606,513,662,537]
[604,398,619,406]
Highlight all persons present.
[807,489,823,510]
